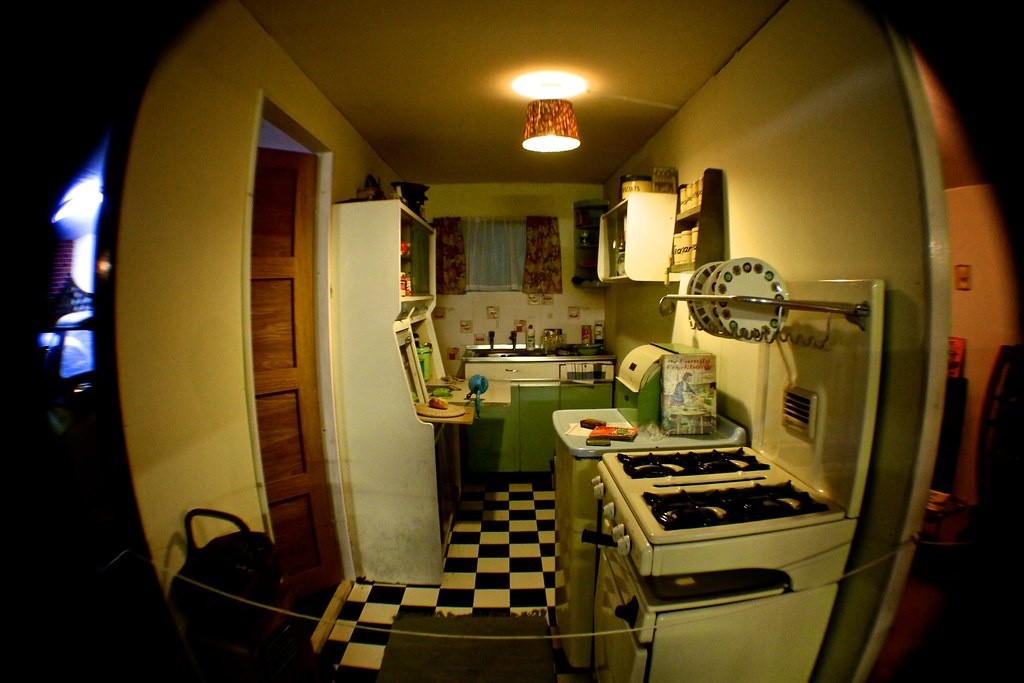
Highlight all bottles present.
[526,325,536,350]
[540,328,563,354]
[617,235,626,276]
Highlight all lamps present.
[522,99,582,152]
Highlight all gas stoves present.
[590,447,845,576]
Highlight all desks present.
[907,490,977,579]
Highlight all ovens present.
[594,514,837,682]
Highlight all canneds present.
[582,325,591,344]
[679,177,703,212]
[674,227,699,265]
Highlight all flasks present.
[417,346,430,380]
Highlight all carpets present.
[378,612,558,683]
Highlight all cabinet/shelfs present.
[465,362,615,474]
[332,200,463,589]
[572,168,723,285]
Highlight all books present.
[650,342,713,355]
[589,426,638,440]
[661,354,717,434]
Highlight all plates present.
[687,257,790,339]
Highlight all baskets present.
[172,509,279,623]
